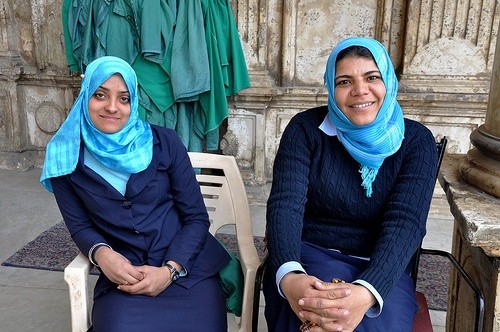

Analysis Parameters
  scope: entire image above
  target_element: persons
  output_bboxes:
[40,57,233,331]
[261,38,440,332]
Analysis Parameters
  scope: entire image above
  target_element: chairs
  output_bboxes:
[251,137,485,332]
[62,152,262,332]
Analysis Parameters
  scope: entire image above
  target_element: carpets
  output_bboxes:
[0,219,452,312]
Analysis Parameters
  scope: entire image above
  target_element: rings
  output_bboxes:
[123,281,128,285]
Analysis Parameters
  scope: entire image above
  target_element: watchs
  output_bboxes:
[164,263,180,281]
